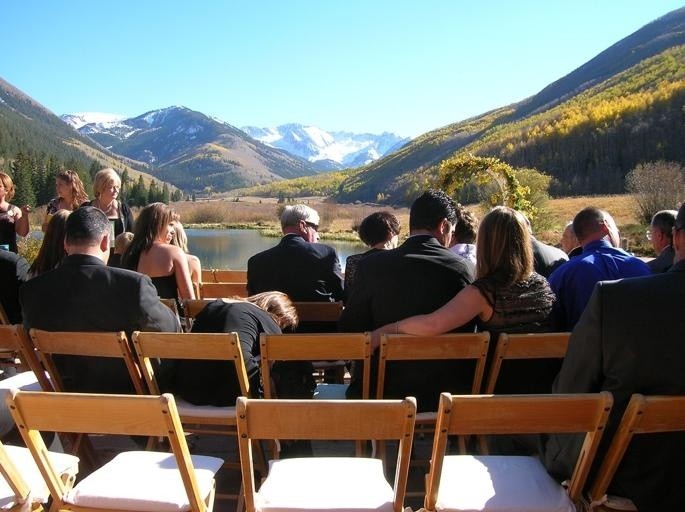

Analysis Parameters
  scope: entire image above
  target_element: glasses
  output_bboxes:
[645,230,656,240]
[305,222,318,232]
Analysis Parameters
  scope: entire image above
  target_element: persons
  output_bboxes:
[344,188,568,453]
[539,203,684,512]
[1,168,202,444]
[247,203,346,384]
[155,290,300,466]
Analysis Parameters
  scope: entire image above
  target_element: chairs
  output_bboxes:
[1,268,685,512]
